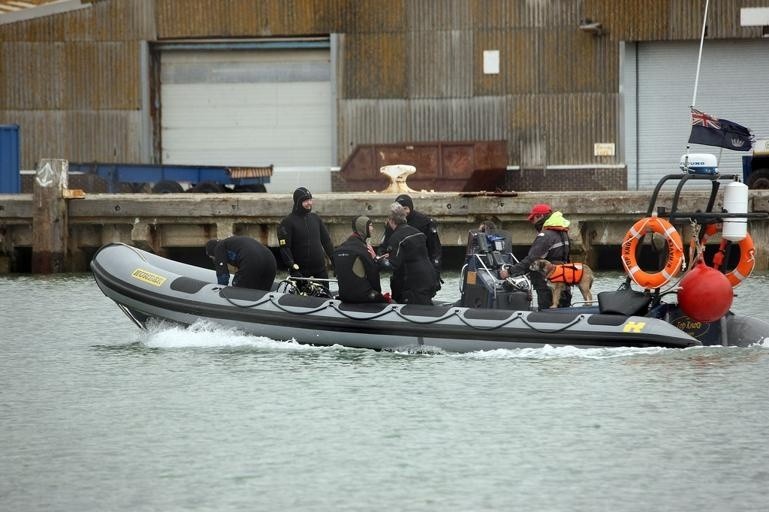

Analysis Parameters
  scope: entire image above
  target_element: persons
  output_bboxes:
[365,201,441,306]
[332,213,397,304]
[206,235,278,292]
[500,204,574,309]
[380,192,443,291]
[277,185,337,297]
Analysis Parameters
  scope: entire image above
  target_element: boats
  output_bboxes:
[89,240,768,358]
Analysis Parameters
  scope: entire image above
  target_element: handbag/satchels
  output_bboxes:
[598,290,651,316]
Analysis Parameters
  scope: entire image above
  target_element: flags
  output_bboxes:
[689,106,753,154]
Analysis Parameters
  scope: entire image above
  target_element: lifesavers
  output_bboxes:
[689,223,756,287]
[622,217,683,289]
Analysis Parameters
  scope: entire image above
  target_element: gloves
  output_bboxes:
[290,268,303,284]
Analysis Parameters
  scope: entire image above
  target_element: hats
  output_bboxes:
[293,188,312,213]
[352,215,370,239]
[395,195,413,213]
[205,241,217,256]
[528,205,552,219]
